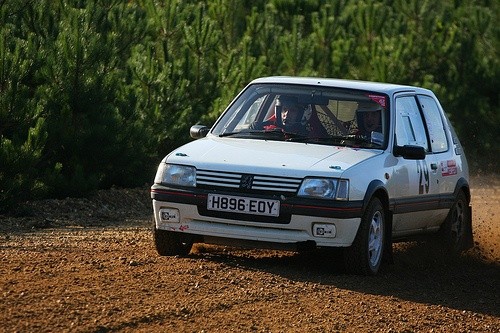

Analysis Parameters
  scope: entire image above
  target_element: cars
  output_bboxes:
[151,75,476,274]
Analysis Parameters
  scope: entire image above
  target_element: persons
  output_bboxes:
[249,94,305,140]
[347,101,381,142]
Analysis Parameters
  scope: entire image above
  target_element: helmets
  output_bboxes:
[356,101,382,112]
[274,95,312,127]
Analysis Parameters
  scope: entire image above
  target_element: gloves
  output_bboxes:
[248,121,264,135]
